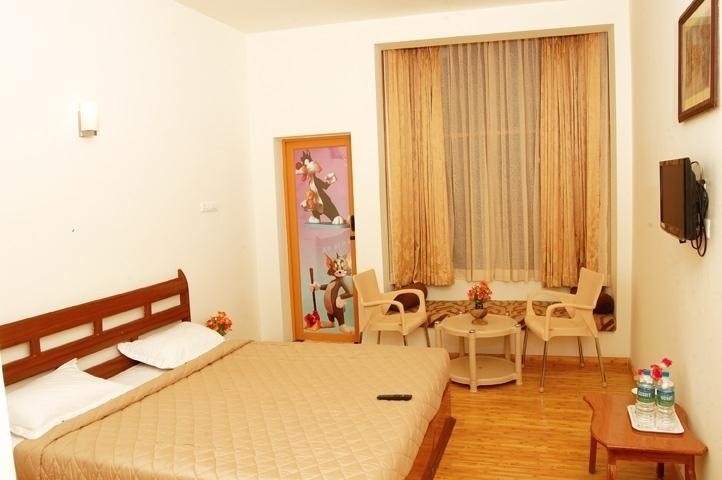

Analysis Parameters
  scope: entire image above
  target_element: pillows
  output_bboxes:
[6,358,129,441]
[117,321,226,369]
[390,281,428,312]
[571,285,614,315]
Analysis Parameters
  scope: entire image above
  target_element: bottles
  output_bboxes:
[635,368,654,427]
[656,371,675,432]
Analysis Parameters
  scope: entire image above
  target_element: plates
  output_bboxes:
[630,387,659,396]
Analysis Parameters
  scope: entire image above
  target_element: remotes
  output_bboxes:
[376,394,413,401]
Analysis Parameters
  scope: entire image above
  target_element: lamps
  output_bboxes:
[76,98,100,140]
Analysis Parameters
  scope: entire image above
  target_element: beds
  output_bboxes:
[1,268,456,480]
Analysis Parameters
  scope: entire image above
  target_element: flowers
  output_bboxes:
[464,278,493,304]
[636,356,672,381]
[205,310,233,337]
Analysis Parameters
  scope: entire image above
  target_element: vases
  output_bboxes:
[470,304,488,325]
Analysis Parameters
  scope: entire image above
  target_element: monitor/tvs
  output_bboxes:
[659,157,701,242]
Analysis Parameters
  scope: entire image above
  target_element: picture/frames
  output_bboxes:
[677,0,718,123]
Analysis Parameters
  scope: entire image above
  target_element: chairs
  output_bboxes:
[352,268,431,349]
[521,267,607,393]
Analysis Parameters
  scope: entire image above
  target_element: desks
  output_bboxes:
[582,392,708,480]
[433,313,523,393]
